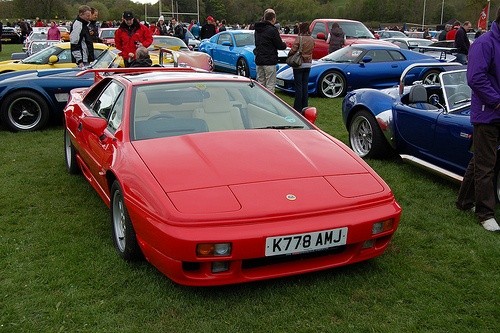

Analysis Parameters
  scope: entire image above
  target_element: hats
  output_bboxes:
[123,11,134,20]
[206,16,213,20]
[158,15,164,21]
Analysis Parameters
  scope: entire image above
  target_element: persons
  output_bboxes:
[367,18,472,65]
[454,7,500,232]
[135,14,254,45]
[326,23,347,54]
[130,46,152,67]
[61,20,73,26]
[252,8,287,94]
[0,16,44,52]
[97,19,122,28]
[114,11,154,68]
[287,22,314,114]
[46,21,61,41]
[69,5,95,67]
[86,7,101,42]
[275,22,300,33]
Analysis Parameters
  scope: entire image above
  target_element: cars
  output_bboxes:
[197,30,289,80]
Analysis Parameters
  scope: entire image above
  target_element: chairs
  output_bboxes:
[409,84,439,111]
[198,87,244,132]
[113,88,154,130]
[453,82,472,101]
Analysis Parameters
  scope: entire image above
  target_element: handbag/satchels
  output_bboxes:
[286,52,302,67]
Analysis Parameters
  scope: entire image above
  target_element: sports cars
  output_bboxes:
[0,22,214,133]
[341,64,475,183]
[275,31,476,100]
[62,70,401,288]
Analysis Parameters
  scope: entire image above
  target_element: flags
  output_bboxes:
[475,3,488,30]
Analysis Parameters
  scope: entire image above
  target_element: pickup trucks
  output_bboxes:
[279,19,400,62]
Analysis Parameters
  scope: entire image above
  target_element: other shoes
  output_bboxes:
[470,206,475,212]
[481,218,500,232]
